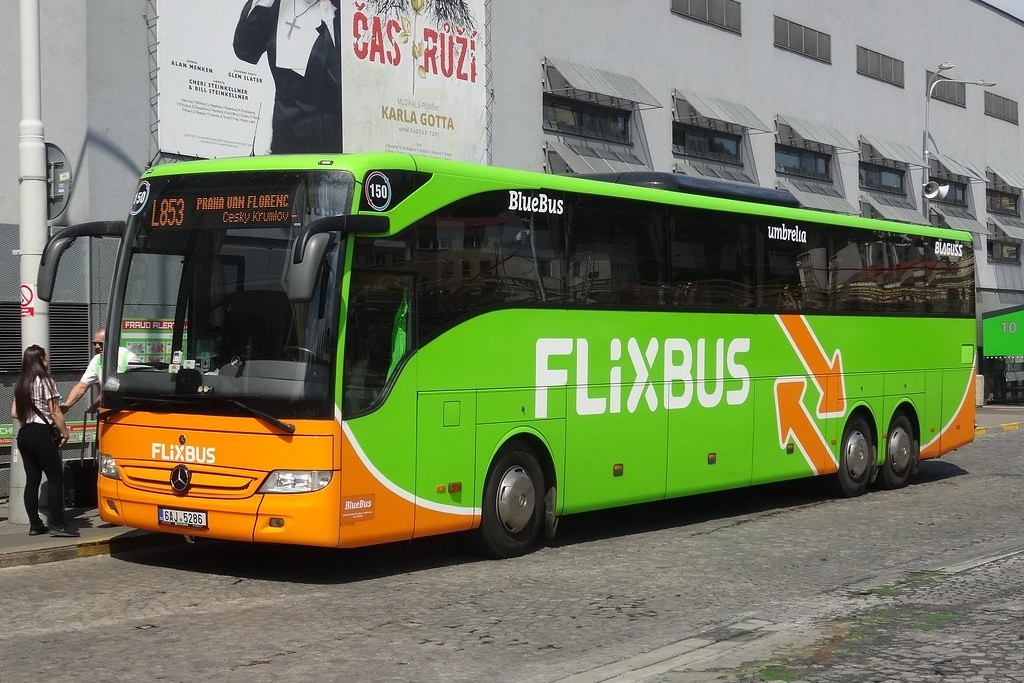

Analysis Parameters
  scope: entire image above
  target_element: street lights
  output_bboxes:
[924,61,997,227]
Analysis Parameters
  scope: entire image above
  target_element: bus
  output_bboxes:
[37,154,977,561]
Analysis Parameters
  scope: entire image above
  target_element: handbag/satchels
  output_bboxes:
[52,427,61,444]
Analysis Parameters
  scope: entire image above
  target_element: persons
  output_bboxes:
[10,344,81,537]
[58,325,144,414]
[233,0,343,155]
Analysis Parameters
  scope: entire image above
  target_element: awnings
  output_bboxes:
[778,177,861,214]
[986,165,1024,190]
[863,136,928,169]
[542,54,663,112]
[931,152,991,182]
[776,113,860,154]
[674,160,759,187]
[672,89,772,137]
[862,193,933,226]
[987,212,1024,239]
[935,206,993,234]
[544,139,652,174]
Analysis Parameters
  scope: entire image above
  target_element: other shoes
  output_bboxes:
[28,521,48,535]
[49,524,80,537]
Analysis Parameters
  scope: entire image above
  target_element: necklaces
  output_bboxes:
[285,0,320,39]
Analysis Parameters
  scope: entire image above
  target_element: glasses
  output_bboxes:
[92,340,103,347]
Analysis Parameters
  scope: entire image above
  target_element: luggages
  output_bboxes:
[64,410,99,508]
